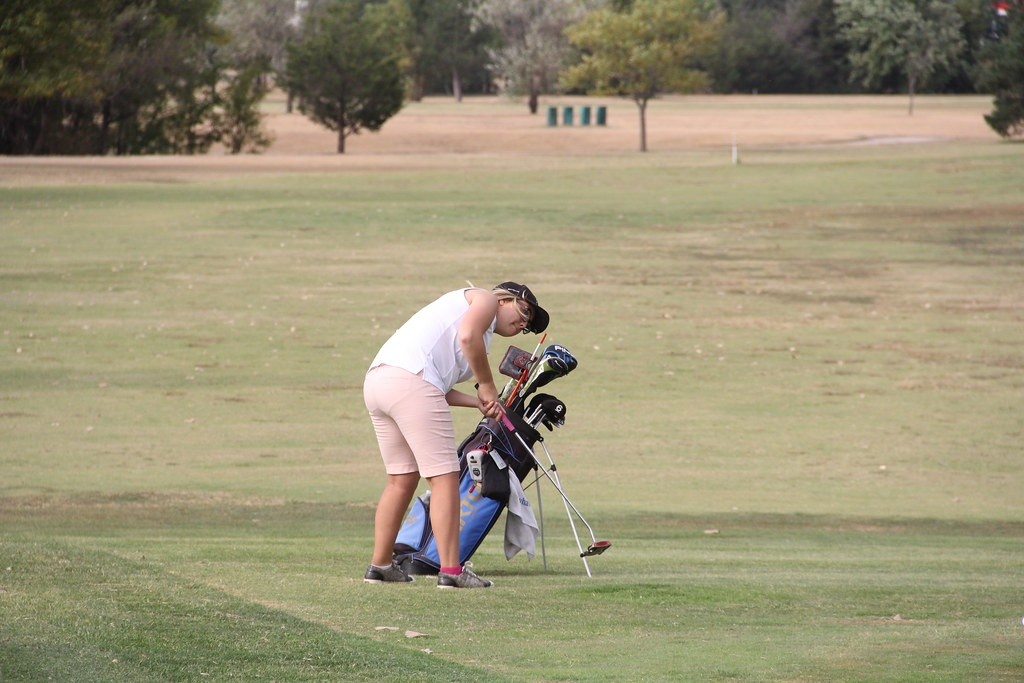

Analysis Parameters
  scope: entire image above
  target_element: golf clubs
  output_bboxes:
[474,381,612,558]
[517,344,580,432]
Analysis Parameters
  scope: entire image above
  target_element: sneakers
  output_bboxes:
[437,561,493,589]
[365,560,413,583]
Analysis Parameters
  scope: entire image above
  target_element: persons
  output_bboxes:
[364,281,549,589]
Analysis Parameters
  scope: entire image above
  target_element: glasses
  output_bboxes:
[516,300,532,334]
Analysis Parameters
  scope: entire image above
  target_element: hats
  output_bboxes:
[494,282,550,333]
